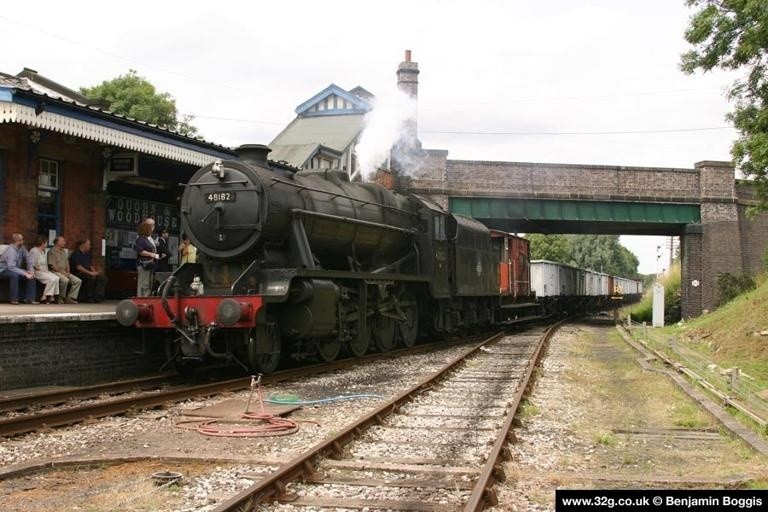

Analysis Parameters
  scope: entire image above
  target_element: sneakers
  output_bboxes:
[10,300,19,305]
[39,296,78,304]
[24,297,41,305]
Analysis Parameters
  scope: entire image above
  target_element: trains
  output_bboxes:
[117,144,645,375]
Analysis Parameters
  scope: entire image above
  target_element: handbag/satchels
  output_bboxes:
[142,259,156,270]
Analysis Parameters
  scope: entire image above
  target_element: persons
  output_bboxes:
[178,233,197,266]
[48,237,82,303]
[70,238,109,303]
[133,217,173,297]
[0,233,40,305]
[29,234,60,304]
